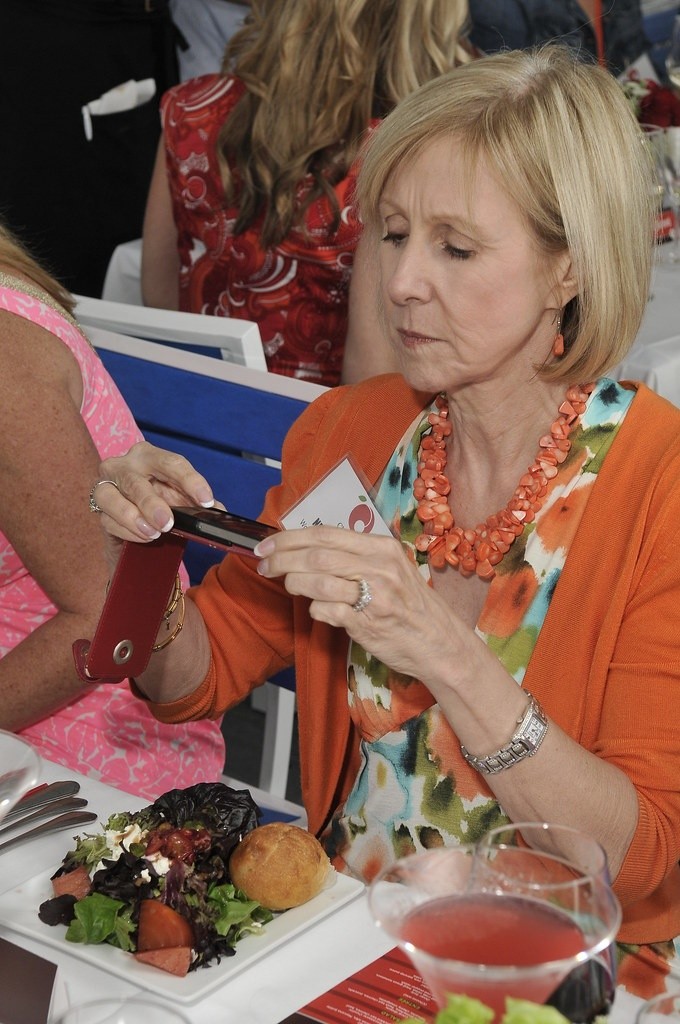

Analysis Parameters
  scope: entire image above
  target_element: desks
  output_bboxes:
[0,750,679,1024]
[98,204,677,406]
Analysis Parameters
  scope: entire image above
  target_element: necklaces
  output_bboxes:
[413,381,593,578]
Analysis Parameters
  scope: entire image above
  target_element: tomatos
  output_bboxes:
[135,947,191,978]
[52,866,92,900]
[137,898,194,952]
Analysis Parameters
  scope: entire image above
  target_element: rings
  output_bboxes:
[89,480,117,513]
[352,580,372,612]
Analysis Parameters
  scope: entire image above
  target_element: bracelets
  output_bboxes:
[104,574,185,652]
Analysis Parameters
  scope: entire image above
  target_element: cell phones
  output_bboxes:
[167,504,280,554]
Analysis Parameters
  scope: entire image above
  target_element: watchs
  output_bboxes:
[459,689,549,774]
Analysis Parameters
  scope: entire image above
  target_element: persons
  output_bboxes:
[90,47,680,958]
[143,0,476,387]
[473,0,680,92]
[0,229,226,1024]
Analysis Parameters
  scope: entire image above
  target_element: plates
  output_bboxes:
[1,852,366,1004]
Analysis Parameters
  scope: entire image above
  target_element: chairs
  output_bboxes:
[84,328,347,824]
[62,290,269,371]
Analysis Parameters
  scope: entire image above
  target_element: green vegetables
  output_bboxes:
[38,782,274,971]
[389,991,576,1024]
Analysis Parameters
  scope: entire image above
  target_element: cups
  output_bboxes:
[369,844,621,1014]
[468,820,618,1022]
[640,125,667,209]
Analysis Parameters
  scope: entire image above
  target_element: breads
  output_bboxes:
[229,822,332,913]
